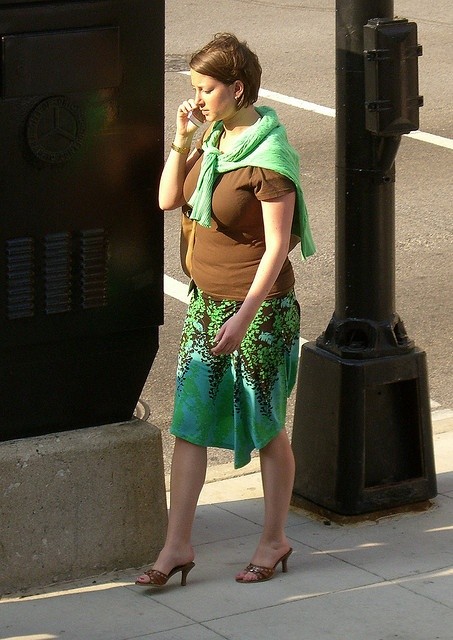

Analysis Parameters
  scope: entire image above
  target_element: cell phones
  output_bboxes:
[188,108,206,128]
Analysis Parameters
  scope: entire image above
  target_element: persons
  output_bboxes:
[135,32,317,587]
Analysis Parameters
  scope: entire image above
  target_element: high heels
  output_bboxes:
[135,562,195,587]
[235,547,293,583]
